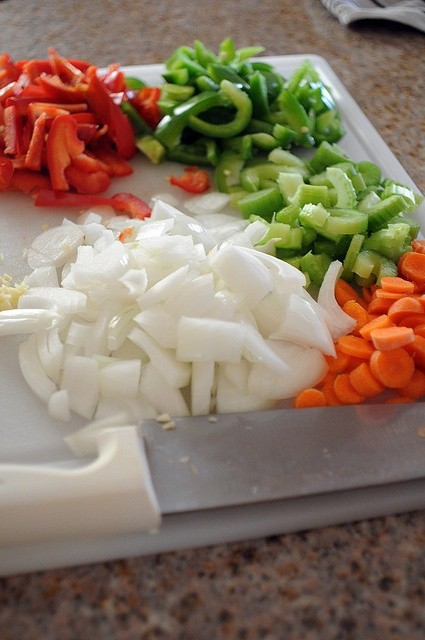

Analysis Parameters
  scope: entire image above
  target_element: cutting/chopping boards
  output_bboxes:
[0,53,423,582]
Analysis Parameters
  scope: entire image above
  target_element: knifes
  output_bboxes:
[1,403,424,525]
